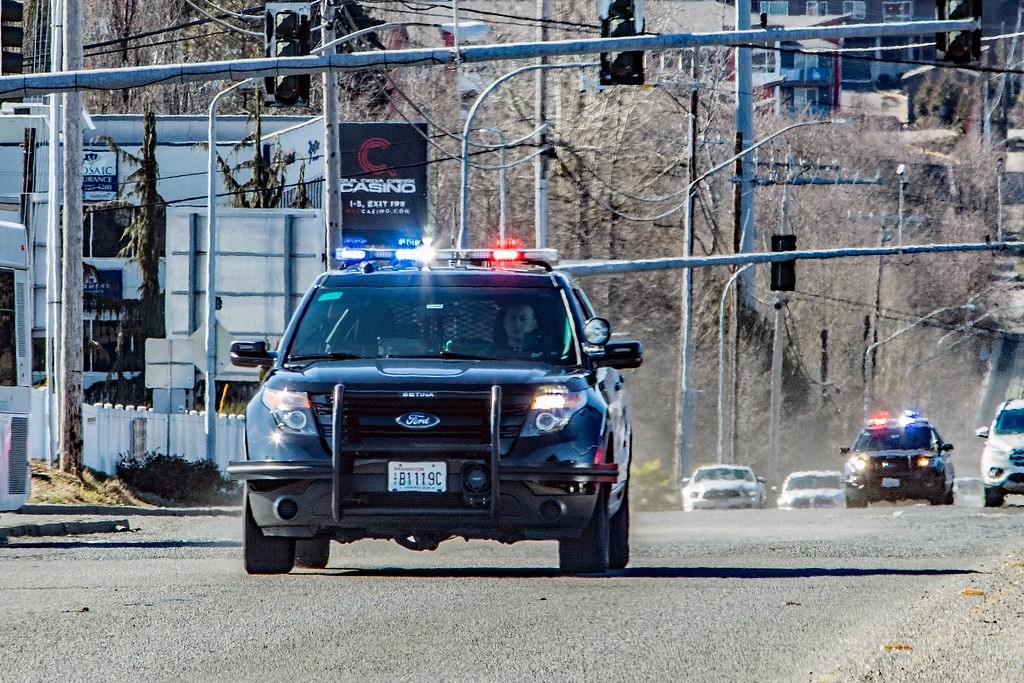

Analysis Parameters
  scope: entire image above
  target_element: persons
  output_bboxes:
[490,302,555,356]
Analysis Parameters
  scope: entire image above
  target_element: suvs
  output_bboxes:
[838,410,957,508]
[975,397,1024,508]
[225,245,643,578]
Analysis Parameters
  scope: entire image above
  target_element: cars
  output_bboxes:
[771,470,848,509]
[680,464,767,511]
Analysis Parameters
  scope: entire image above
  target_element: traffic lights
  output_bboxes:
[1,0,25,75]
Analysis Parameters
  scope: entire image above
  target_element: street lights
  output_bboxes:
[455,62,697,252]
[669,116,850,493]
[197,20,492,461]
